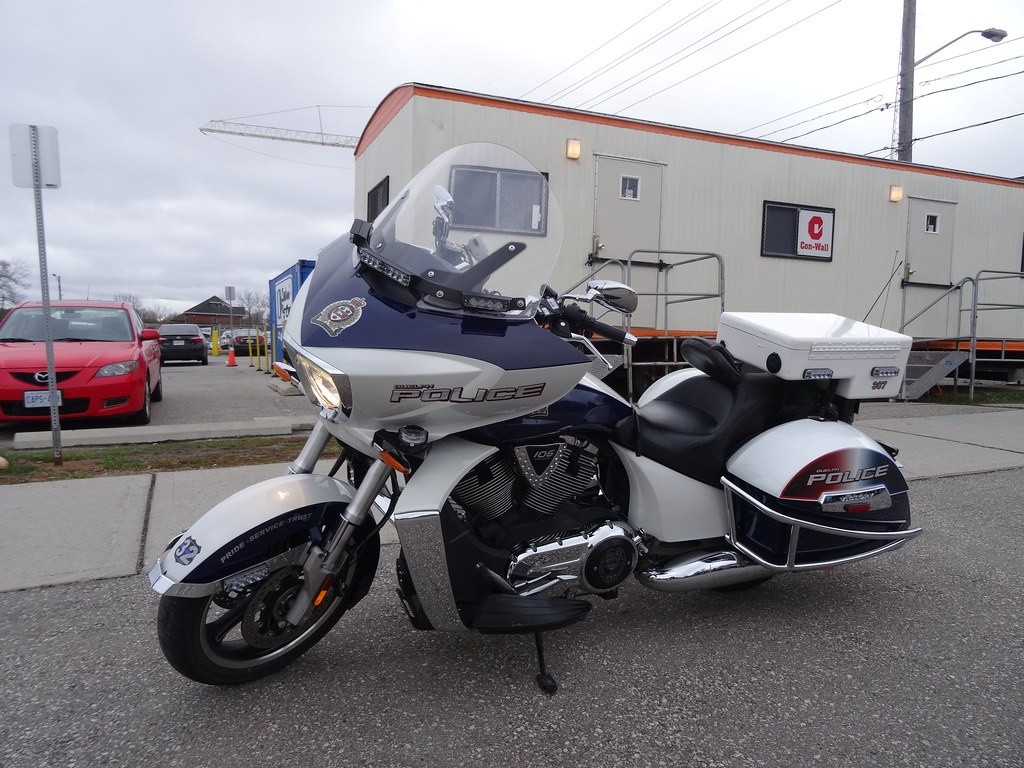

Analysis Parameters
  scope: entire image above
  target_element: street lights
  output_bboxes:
[896,0,1006,164]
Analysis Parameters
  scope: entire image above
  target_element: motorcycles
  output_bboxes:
[147,142,923,685]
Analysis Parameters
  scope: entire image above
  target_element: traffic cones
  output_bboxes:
[226,346,237,366]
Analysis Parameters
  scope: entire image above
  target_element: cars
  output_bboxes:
[219,329,272,357]
[0,300,164,424]
[157,323,212,366]
